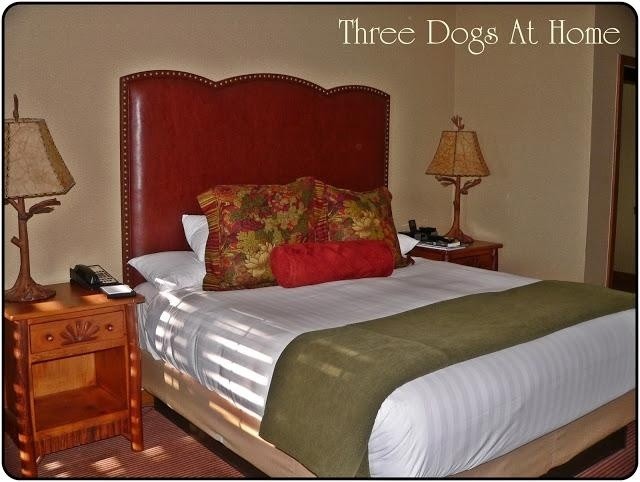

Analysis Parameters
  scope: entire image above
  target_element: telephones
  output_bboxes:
[70,264,122,290]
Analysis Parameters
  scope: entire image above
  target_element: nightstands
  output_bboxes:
[405,240,503,271]
[4,281,145,478]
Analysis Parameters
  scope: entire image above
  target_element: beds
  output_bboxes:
[134,252,635,478]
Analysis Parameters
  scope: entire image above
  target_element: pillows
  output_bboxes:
[127,178,421,293]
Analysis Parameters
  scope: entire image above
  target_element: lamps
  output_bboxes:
[4,93,76,303]
[425,115,490,244]
